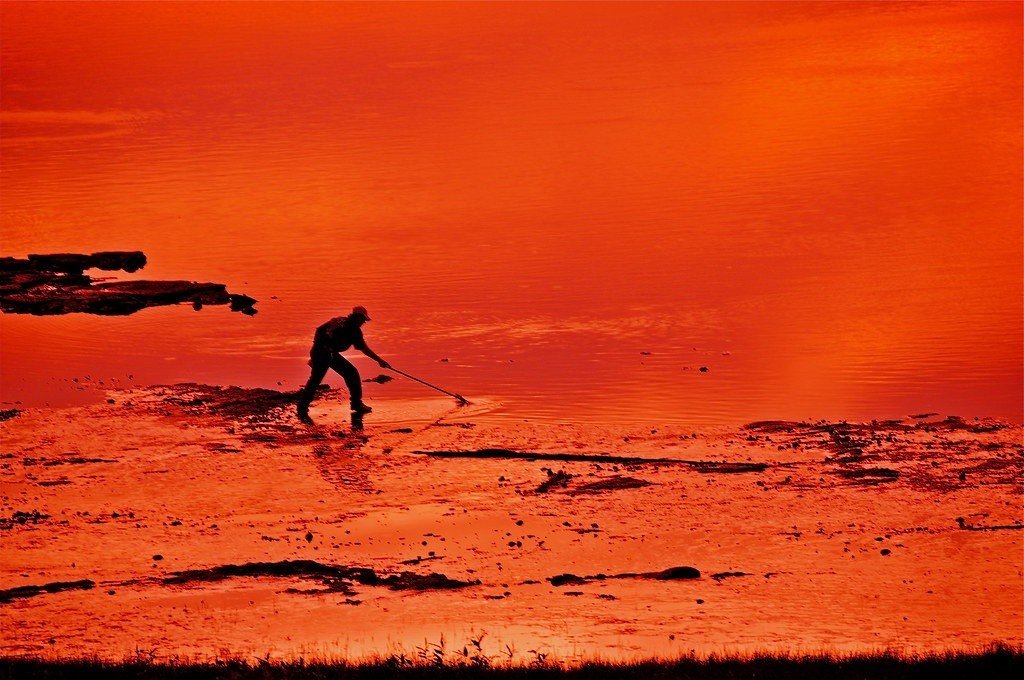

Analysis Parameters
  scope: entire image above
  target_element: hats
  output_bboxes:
[353,305,372,321]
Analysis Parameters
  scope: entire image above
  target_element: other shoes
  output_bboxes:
[298,410,310,420]
[351,402,372,411]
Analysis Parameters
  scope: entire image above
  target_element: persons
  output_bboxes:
[296,305,390,426]
